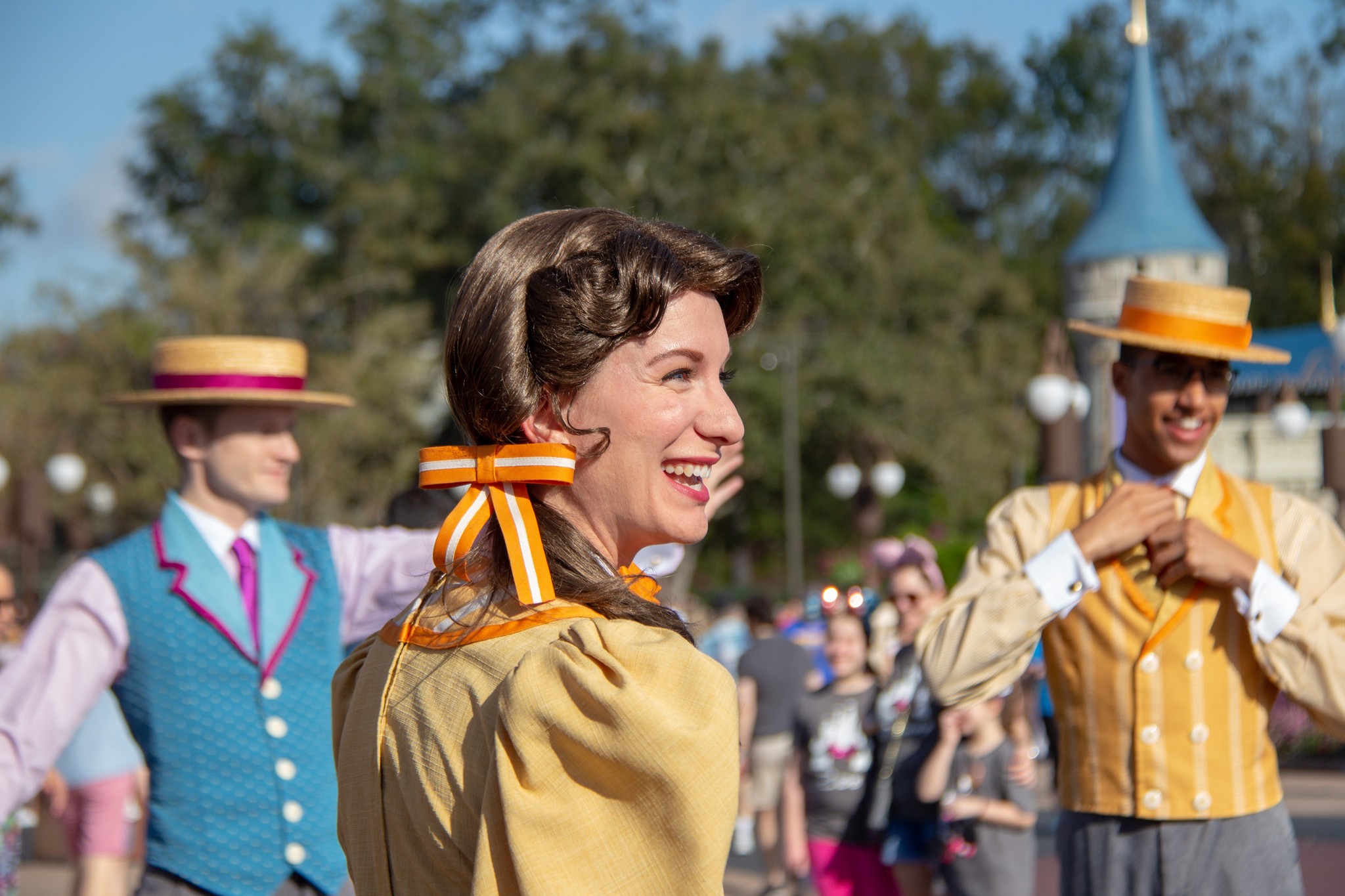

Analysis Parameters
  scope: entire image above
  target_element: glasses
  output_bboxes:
[1124,354,1239,395]
[0,597,17,608]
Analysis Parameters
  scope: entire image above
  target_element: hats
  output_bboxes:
[103,337,356,409]
[1067,275,1291,364]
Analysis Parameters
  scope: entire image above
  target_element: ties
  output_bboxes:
[234,538,260,650]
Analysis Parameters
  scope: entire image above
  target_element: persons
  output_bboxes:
[332,207,762,894]
[693,531,1059,896]
[0,565,140,896]
[913,278,1345,896]
[0,335,744,896]
